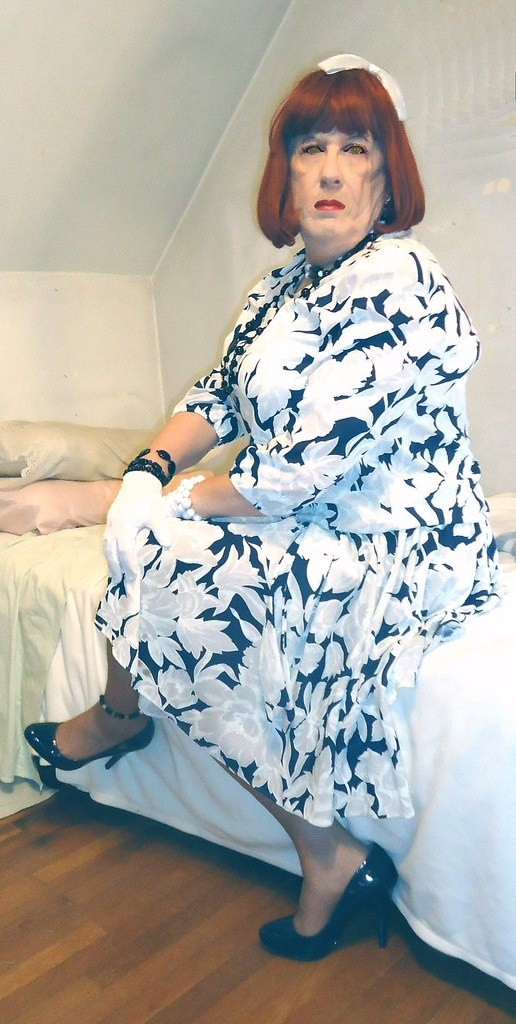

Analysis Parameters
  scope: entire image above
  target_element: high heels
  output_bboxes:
[258,842,398,960]
[25,715,154,770]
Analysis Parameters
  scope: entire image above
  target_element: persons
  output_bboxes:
[25,53,498,959]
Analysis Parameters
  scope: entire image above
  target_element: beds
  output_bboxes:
[0,491,515,994]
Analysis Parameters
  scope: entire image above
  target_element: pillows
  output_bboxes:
[0,480,124,538]
[0,420,154,481]
